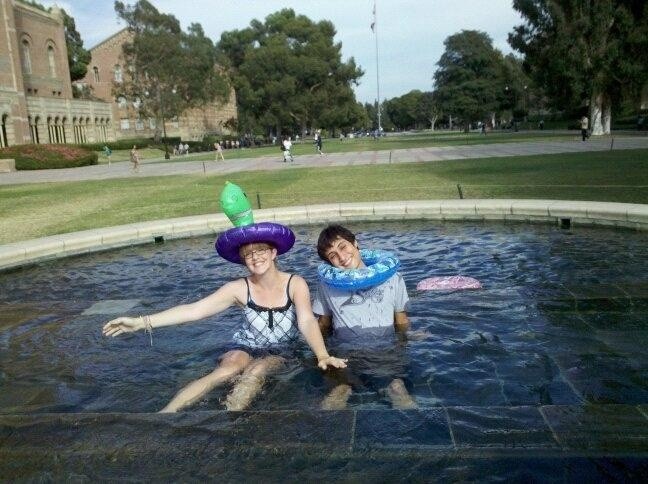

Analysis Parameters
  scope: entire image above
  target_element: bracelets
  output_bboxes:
[138,312,155,348]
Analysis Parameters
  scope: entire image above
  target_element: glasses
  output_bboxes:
[240,246,272,259]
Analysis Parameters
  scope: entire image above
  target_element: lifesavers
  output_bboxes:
[316,249,401,291]
[417,275,482,292]
[215,181,296,265]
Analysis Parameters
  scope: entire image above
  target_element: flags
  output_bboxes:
[371,7,375,33]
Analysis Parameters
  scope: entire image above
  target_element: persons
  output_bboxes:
[212,142,224,162]
[312,132,325,156]
[127,143,143,173]
[220,137,245,152]
[280,136,295,162]
[101,145,113,167]
[310,222,431,413]
[99,236,350,415]
[171,141,191,157]
[580,113,592,141]
